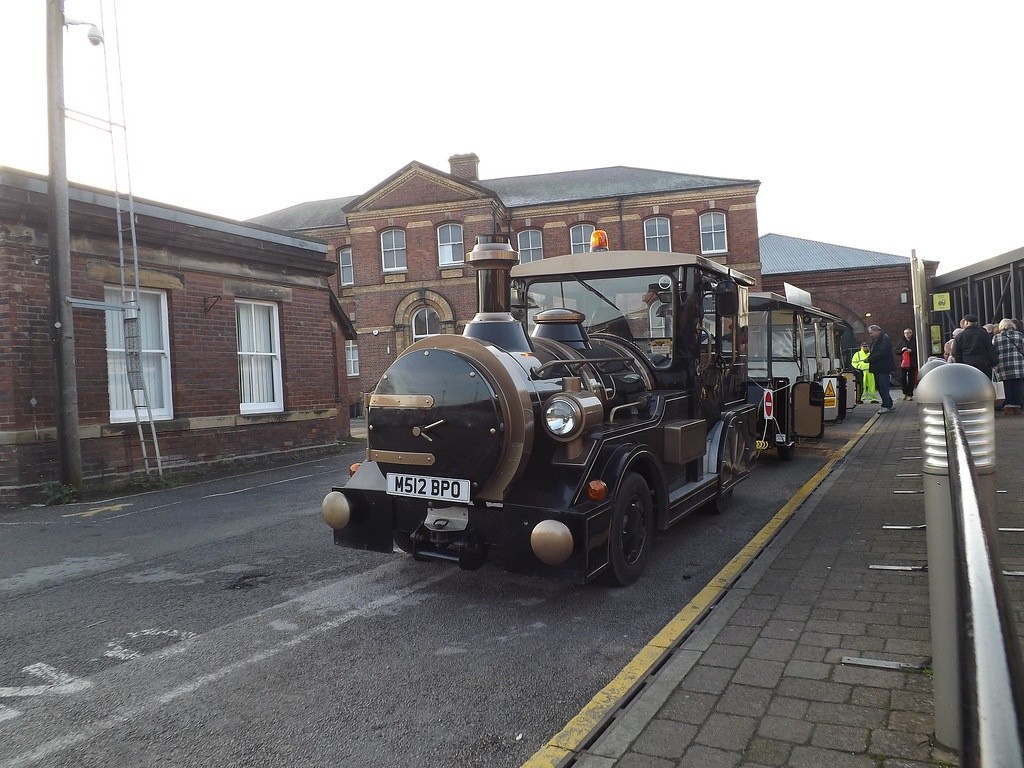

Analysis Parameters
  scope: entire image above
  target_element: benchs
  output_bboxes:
[747,354,840,403]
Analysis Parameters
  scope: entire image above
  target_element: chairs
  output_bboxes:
[583,306,634,343]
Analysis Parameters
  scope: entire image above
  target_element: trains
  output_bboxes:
[322,229,865,590]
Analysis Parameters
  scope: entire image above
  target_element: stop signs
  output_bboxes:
[765,391,774,418]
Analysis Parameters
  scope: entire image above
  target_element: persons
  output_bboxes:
[863,325,895,414]
[851,341,879,402]
[894,327,917,401]
[942,315,1024,414]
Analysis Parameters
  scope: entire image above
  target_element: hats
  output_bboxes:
[964,314,978,322]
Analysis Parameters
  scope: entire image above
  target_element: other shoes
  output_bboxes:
[902,394,906,400]
[1002,404,1013,415]
[858,401,863,404]
[906,396,913,401]
[877,407,889,414]
[871,400,879,403]
[1013,405,1021,414]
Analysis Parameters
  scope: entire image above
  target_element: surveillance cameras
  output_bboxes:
[88,27,103,46]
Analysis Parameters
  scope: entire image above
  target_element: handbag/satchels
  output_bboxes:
[991,369,1006,400]
[901,352,910,368]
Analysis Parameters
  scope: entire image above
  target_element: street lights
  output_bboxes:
[45,0,107,488]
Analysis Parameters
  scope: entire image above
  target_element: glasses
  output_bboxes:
[864,346,867,347]
